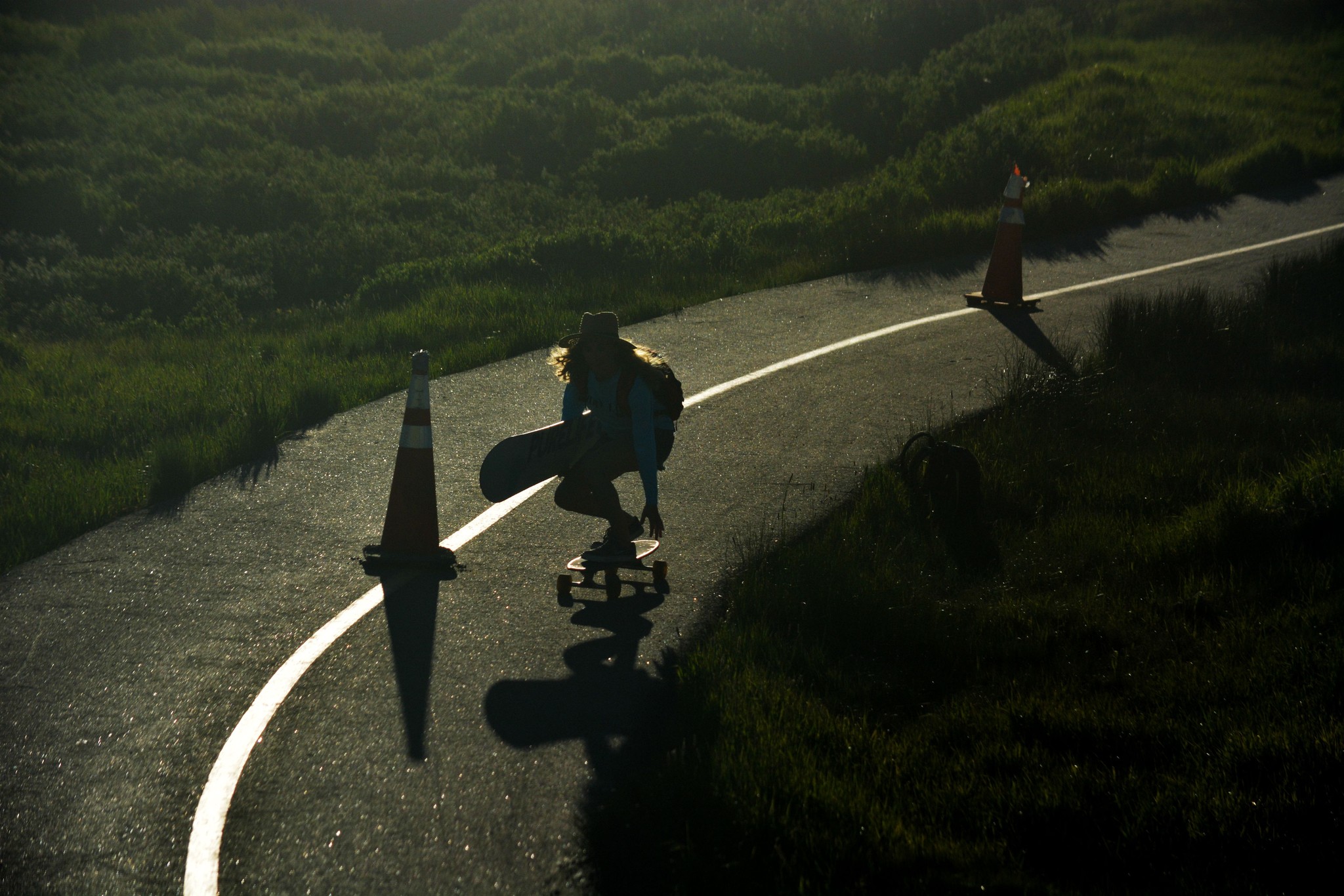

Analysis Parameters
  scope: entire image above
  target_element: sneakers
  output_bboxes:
[582,539,636,562]
[590,516,644,549]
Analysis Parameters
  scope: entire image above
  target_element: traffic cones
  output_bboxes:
[964,161,1043,316]
[349,349,462,576]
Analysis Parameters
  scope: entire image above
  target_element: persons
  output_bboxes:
[553,312,684,555]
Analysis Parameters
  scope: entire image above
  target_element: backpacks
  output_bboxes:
[576,352,684,422]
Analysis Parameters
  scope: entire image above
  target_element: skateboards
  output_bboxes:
[555,538,668,597]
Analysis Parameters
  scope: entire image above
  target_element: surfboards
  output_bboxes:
[479,408,599,504]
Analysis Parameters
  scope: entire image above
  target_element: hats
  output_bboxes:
[558,312,637,352]
